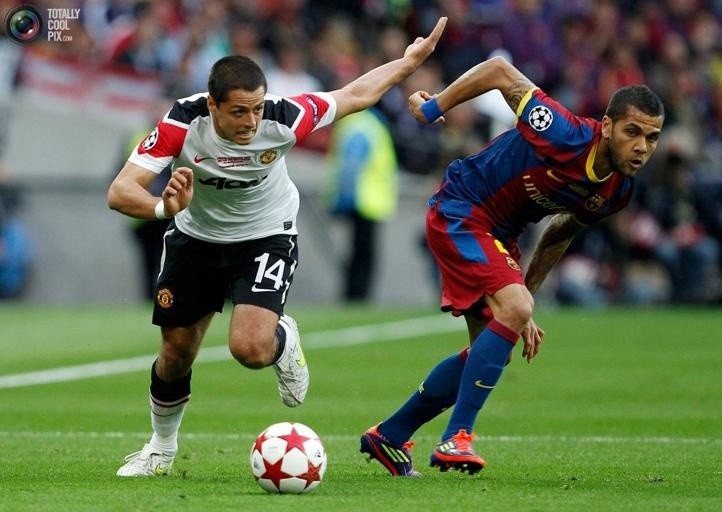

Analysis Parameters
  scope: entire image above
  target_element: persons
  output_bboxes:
[65,16,452,490]
[316,102,400,304]
[0,0,720,312]
[359,52,669,479]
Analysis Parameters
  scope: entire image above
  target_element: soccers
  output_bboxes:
[250,421,327,494]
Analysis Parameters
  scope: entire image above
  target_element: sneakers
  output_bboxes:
[359,422,423,479]
[429,428,488,475]
[272,314,310,408]
[115,443,174,477]
